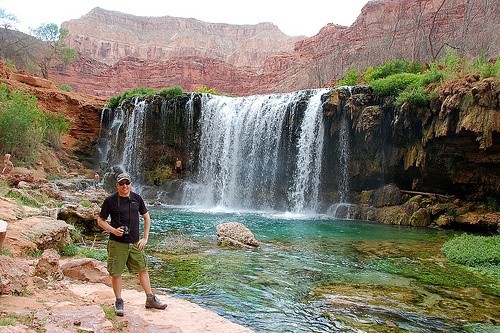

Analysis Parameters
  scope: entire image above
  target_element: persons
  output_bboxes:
[93,172,100,189]
[97,172,167,316]
[175,157,181,179]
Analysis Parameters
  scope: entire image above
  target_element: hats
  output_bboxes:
[117,173,130,182]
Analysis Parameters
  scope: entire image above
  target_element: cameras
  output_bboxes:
[119,226,131,238]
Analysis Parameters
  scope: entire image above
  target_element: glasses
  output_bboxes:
[118,181,130,186]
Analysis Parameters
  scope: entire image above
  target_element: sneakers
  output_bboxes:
[115,298,124,315]
[145,294,168,309]
